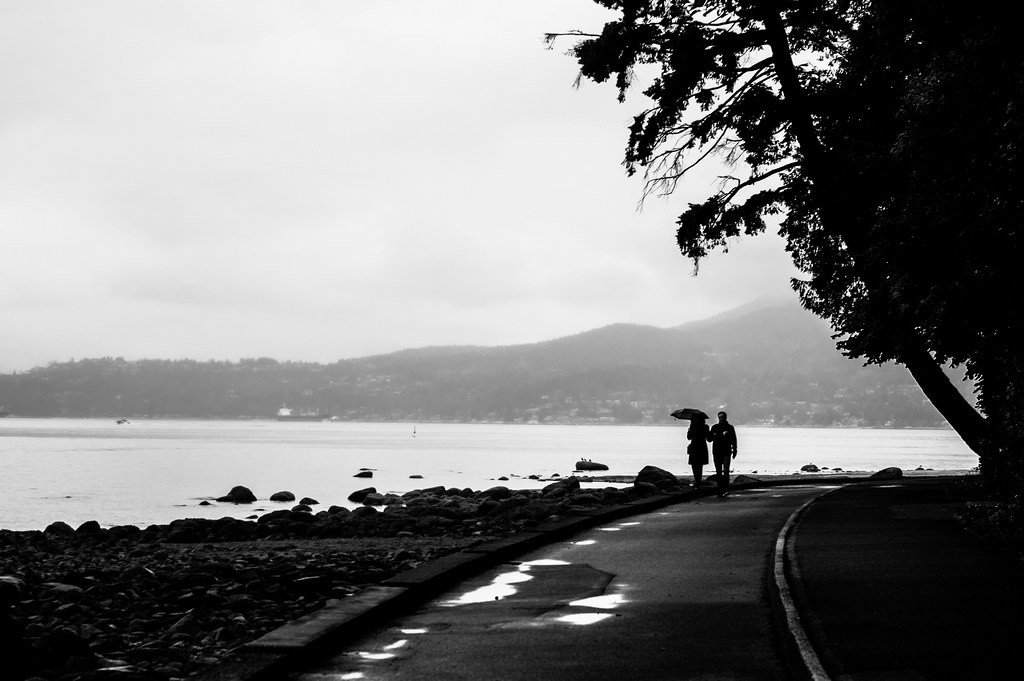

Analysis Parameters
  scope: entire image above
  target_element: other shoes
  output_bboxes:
[717,490,730,498]
[694,482,700,491]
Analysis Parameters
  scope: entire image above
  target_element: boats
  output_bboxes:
[277,408,329,422]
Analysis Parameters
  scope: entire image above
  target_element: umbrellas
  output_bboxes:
[672,408,709,419]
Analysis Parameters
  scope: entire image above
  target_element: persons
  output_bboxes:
[710,411,737,496]
[687,414,716,487]
